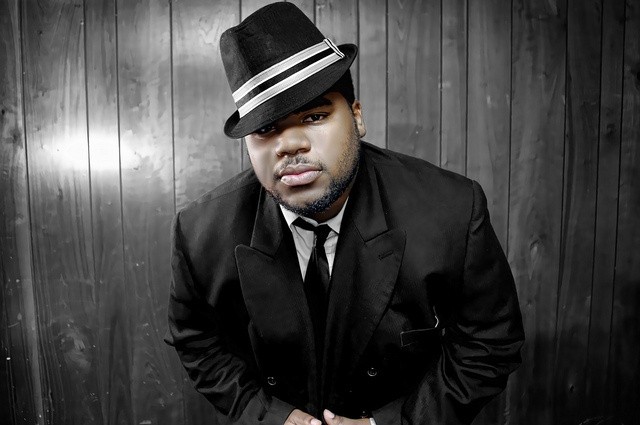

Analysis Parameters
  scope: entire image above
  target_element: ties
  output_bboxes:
[292,217,331,383]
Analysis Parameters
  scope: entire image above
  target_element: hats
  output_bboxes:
[219,1,357,139]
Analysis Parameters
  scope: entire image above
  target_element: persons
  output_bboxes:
[164,2,525,424]
